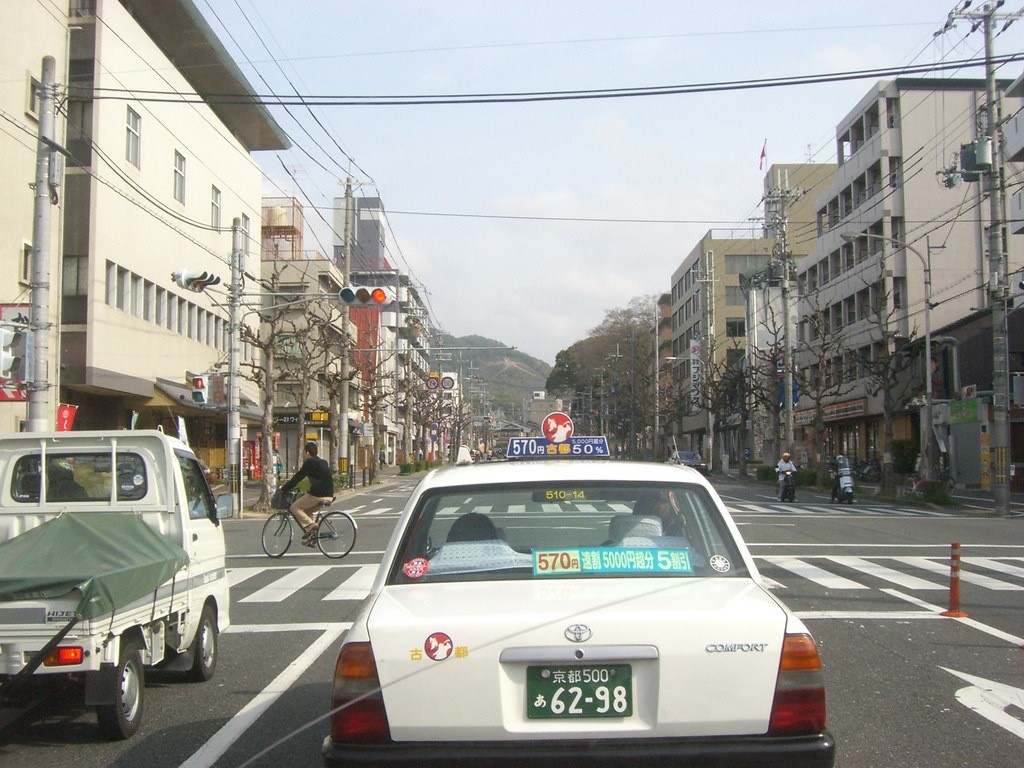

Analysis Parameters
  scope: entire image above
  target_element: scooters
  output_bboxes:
[828,457,855,505]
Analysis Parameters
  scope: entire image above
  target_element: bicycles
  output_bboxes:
[902,467,957,498]
[262,487,358,559]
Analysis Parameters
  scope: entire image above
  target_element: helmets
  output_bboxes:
[836,454,844,464]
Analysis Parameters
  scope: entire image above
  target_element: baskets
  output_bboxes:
[270,490,293,509]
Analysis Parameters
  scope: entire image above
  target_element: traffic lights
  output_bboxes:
[191,374,209,405]
[338,286,393,306]
[171,269,221,294]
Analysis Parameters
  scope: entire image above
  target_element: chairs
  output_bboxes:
[611,536,705,566]
[608,512,667,542]
[116,471,146,499]
[427,537,529,573]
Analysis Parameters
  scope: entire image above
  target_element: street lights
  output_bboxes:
[665,356,713,473]
[841,231,934,478]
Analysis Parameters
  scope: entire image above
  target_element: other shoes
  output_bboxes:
[301,538,317,548]
[302,522,318,539]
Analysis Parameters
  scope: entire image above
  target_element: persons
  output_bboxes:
[448,513,498,541]
[632,490,674,534]
[774,452,798,502]
[278,442,334,547]
[829,452,857,504]
[379,448,386,469]
[275,451,282,479]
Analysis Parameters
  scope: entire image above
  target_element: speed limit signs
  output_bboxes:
[441,376,454,390]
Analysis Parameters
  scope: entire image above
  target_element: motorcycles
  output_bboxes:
[775,463,801,503]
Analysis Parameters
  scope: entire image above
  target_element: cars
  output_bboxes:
[664,451,708,477]
[320,411,837,768]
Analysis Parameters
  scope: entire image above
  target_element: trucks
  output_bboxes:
[1,429,234,741]
